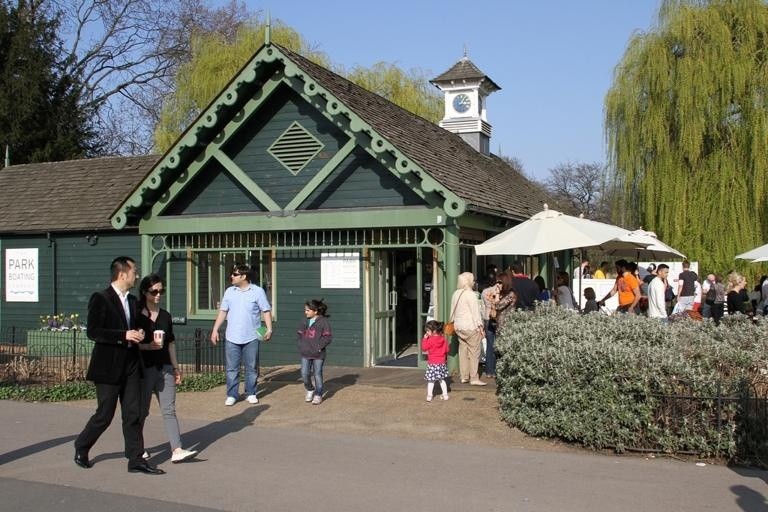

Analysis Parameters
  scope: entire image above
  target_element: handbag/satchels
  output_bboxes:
[705,288,716,305]
[665,284,674,302]
[445,321,455,336]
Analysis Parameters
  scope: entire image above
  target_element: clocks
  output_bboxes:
[453,94,471,113]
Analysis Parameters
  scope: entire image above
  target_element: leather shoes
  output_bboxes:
[75,448,89,468]
[128,459,163,475]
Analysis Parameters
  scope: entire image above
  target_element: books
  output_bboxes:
[255,326,267,338]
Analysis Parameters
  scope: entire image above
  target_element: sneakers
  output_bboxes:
[171,449,198,463]
[312,395,322,404]
[471,380,486,386]
[247,394,258,403]
[305,390,314,401]
[226,397,236,406]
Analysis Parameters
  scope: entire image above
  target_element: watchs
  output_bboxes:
[267,329,272,333]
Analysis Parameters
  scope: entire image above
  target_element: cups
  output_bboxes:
[153,329,165,349]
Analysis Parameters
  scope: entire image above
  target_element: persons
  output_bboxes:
[573,257,640,315]
[481,261,548,378]
[210,263,274,407]
[401,261,433,336]
[450,272,487,386]
[635,263,675,315]
[73,257,165,476]
[134,273,199,464]
[295,298,333,406]
[694,273,726,315]
[553,271,575,309]
[672,259,699,314]
[421,320,449,402]
[727,270,767,315]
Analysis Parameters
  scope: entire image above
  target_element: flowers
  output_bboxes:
[39,314,87,332]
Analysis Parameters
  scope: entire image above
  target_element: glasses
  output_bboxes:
[233,272,241,277]
[148,289,166,296]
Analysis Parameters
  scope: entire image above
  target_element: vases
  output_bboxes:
[27,330,95,357]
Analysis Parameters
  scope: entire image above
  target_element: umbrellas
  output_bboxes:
[474,202,768,315]
